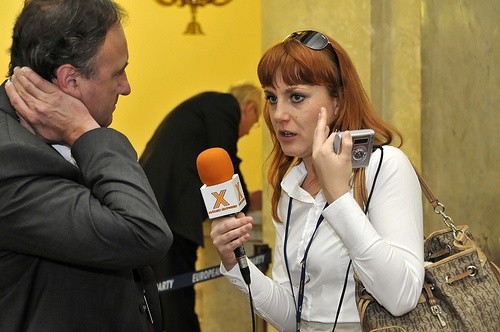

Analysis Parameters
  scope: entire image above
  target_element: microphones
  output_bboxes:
[197,148,252,285]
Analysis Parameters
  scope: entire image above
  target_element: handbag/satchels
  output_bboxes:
[352,144,499,332]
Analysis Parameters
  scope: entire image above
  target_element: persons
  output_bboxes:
[138,84,262,332]
[211,30,425,332]
[0,0,173,332]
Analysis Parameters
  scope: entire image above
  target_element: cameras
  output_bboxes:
[334,129,375,168]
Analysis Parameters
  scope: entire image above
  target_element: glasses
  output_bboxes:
[252,107,260,128]
[281,30,337,55]
